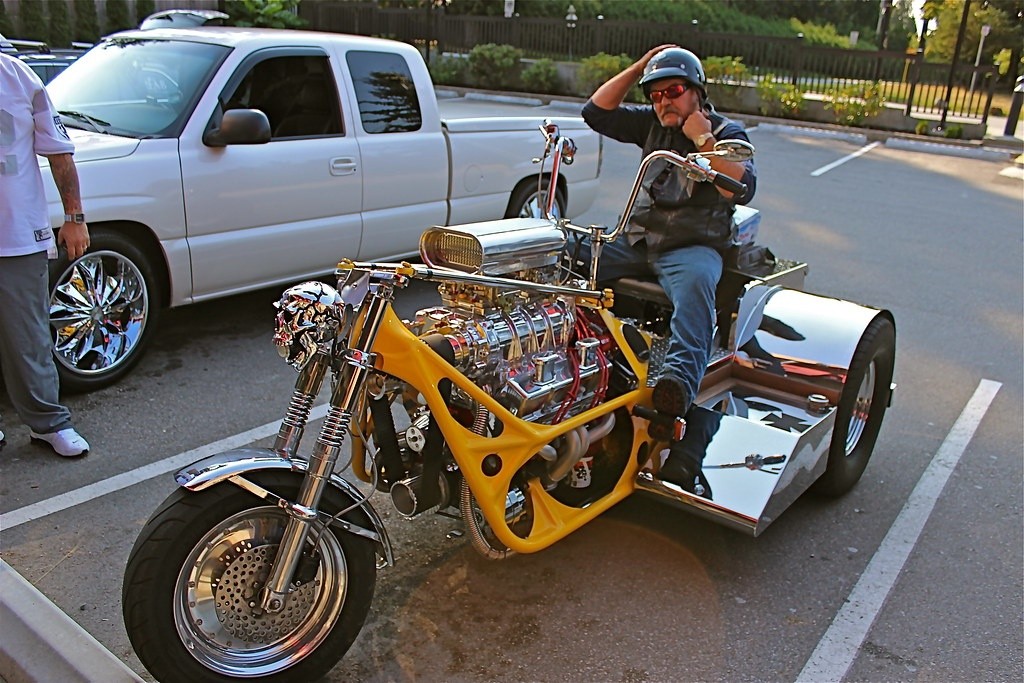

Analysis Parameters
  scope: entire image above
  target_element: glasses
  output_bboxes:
[650,81,692,102]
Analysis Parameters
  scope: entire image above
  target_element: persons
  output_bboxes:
[2,45,96,457]
[548,44,763,446]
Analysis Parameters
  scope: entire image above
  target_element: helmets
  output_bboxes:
[637,46,707,98]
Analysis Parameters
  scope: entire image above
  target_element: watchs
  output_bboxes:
[694,130,714,149]
[63,212,85,225]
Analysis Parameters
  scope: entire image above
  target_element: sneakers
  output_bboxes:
[30,428,90,458]
[647,377,692,442]
[0,428,6,452]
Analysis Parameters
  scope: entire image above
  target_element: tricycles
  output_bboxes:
[120,113,903,683]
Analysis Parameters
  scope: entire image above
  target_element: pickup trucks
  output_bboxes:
[27,25,604,394]
